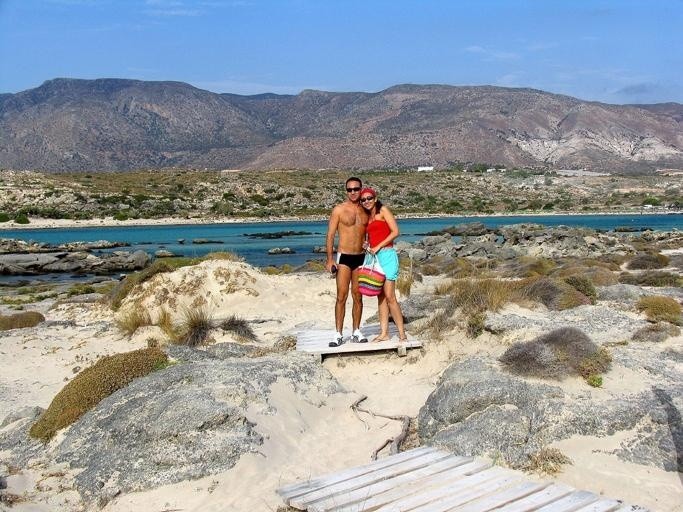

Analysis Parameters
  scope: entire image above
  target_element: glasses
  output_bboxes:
[359,196,373,202]
[346,187,360,192]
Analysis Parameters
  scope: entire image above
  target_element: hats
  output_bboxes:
[359,188,377,197]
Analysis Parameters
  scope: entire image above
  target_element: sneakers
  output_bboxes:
[327,332,343,347]
[349,329,367,343]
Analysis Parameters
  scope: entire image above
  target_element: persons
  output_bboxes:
[323,176,369,348]
[356,187,408,344]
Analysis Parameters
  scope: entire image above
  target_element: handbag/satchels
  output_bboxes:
[355,251,385,298]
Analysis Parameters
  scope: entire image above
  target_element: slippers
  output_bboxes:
[370,334,386,344]
[397,336,408,344]
